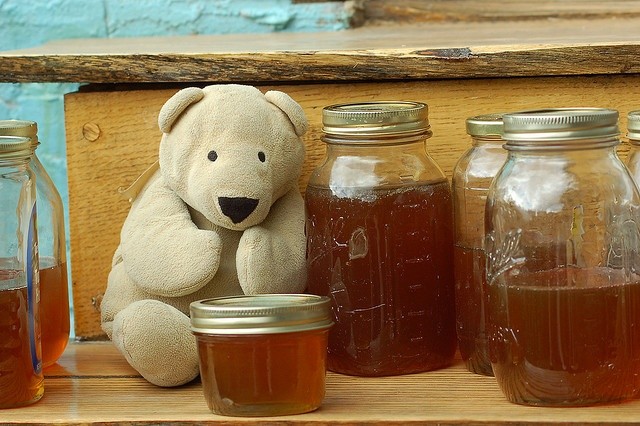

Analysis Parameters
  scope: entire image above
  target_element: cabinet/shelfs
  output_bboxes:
[0,19,640,426]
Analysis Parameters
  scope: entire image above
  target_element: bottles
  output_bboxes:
[481,106,639,406]
[0,136,46,410]
[0,117,70,366]
[299,98,462,375]
[450,106,572,378]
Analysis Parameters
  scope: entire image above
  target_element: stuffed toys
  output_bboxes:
[99,84,313,387]
